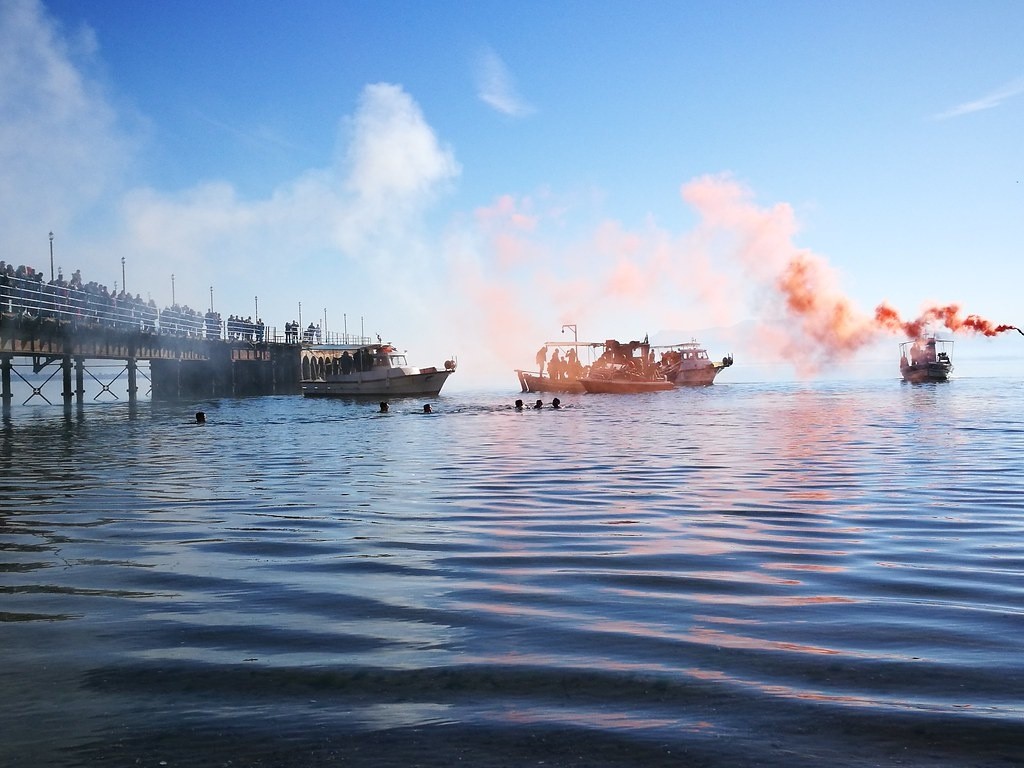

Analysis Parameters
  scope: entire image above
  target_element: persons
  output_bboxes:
[534,400,543,408]
[0,261,158,333]
[552,397,560,408]
[380,402,389,412]
[285,320,299,344]
[424,404,433,413]
[622,349,661,381]
[196,412,207,423]
[515,400,523,408]
[205,308,222,339]
[304,322,322,345]
[660,351,679,366]
[160,304,204,337]
[338,349,374,375]
[536,346,577,379]
[228,314,264,342]
[910,343,918,366]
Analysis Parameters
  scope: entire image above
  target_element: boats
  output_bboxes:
[899,328,952,383]
[578,334,677,392]
[652,338,733,386]
[300,335,456,399]
[514,324,684,394]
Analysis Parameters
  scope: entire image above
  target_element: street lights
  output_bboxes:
[344,313,347,344]
[121,256,127,293]
[210,286,214,313]
[298,302,302,339]
[171,273,175,306]
[361,316,364,345]
[49,230,55,281]
[324,308,327,343]
[255,296,258,324]
